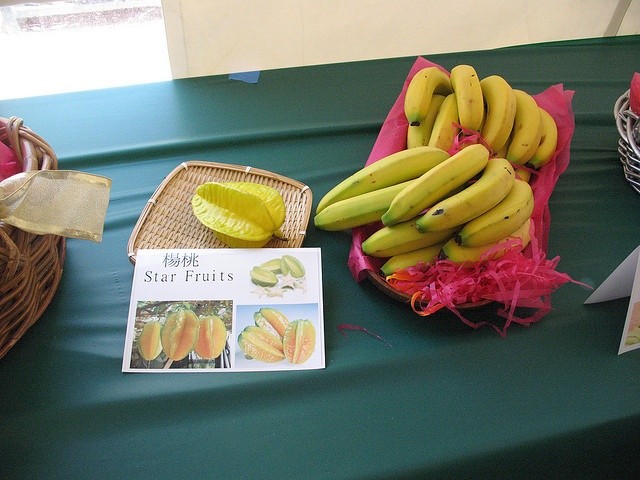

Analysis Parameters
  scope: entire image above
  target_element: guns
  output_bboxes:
[404,65,559,183]
[313,143,535,277]
